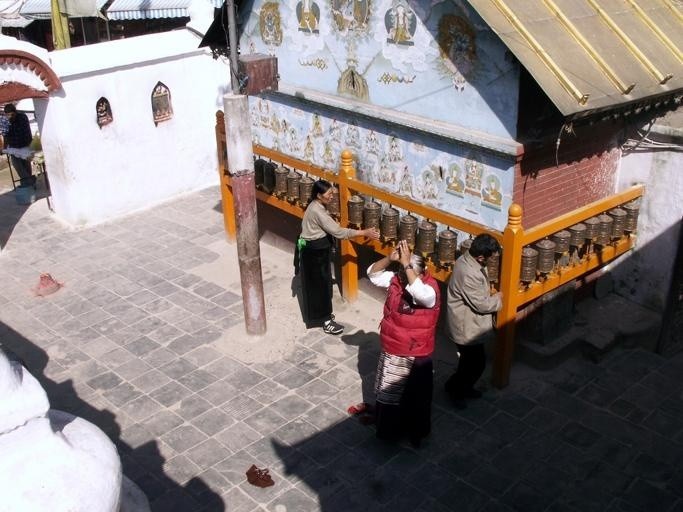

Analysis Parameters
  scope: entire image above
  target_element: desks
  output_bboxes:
[4,147,52,213]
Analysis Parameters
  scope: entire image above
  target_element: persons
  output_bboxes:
[3,103,39,187]
[293,178,379,334]
[367,238,438,449]
[445,233,504,410]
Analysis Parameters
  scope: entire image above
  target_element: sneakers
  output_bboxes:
[322,314,344,334]
